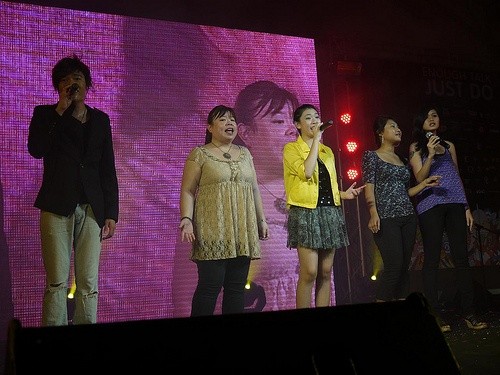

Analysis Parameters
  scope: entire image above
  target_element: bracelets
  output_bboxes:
[180,216,192,222]
[257,220,268,223]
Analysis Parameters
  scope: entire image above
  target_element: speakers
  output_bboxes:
[3,291,465,375]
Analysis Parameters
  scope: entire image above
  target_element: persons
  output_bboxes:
[407,105,488,332]
[27,55,121,326]
[226,80,338,312]
[176,106,269,317]
[284,103,366,309]
[362,116,444,303]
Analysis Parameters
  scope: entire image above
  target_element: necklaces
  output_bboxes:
[210,140,233,159]
[256,179,288,214]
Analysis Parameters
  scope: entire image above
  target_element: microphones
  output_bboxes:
[67,82,79,98]
[425,131,451,149]
[319,119,334,132]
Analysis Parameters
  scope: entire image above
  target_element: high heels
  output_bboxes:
[440,325,451,332]
[464,319,487,329]
